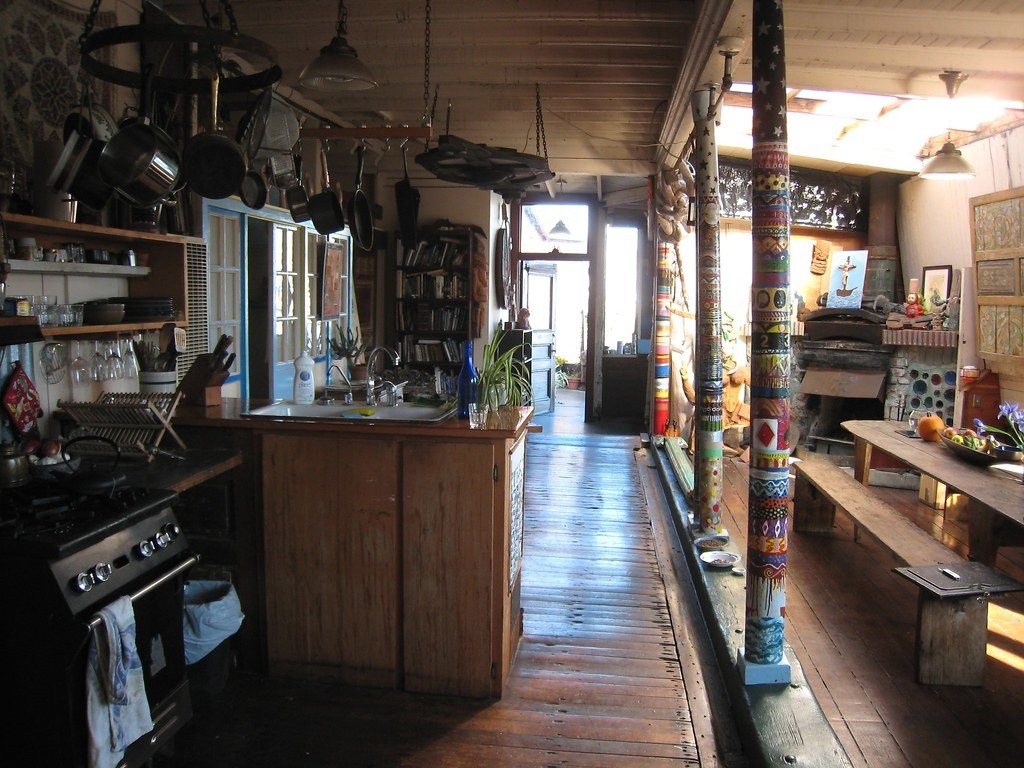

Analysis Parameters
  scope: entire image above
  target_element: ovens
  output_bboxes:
[0,547,195,767]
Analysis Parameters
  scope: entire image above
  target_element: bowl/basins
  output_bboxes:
[993,444,1023,462]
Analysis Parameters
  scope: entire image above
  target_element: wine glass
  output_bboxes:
[70,339,138,382]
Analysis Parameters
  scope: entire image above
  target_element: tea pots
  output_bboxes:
[0,439,31,487]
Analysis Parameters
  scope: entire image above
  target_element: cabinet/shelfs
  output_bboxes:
[0,211,189,344]
[392,224,488,382]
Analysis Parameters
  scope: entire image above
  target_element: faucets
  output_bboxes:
[366,345,400,405]
[327,365,353,404]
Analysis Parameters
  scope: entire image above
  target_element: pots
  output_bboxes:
[30,31,371,247]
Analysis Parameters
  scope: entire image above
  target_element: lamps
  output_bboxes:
[547,177,571,237]
[296,0,379,93]
[917,127,981,180]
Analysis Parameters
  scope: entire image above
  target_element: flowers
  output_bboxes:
[973,401,1024,455]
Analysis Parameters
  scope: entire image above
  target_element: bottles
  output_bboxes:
[293,350,315,405]
[909,409,929,434]
[456,341,479,419]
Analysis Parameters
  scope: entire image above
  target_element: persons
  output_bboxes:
[838,262,855,285]
[680,321,750,458]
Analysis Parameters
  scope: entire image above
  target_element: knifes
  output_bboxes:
[206,335,237,372]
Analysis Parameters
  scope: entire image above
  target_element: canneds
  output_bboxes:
[4,297,29,316]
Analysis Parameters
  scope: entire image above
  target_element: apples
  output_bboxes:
[944,426,989,452]
[22,439,58,456]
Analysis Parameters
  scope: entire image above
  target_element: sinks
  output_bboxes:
[373,406,440,420]
[263,405,348,418]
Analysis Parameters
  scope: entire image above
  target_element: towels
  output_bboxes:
[84,594,154,768]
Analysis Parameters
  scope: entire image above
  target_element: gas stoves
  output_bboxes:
[0,435,189,619]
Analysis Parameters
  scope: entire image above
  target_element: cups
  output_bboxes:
[467,403,489,430]
[10,294,85,329]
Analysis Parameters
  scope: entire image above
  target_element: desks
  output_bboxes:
[841,419,1024,574]
[240,405,536,700]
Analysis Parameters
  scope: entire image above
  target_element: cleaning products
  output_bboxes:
[293,351,315,404]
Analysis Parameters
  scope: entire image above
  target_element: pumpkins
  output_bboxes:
[918,411,945,442]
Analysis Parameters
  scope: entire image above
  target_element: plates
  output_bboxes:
[936,428,1006,465]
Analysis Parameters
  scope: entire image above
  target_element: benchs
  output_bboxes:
[793,460,988,687]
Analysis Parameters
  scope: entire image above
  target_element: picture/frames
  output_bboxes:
[921,265,954,312]
[319,241,343,318]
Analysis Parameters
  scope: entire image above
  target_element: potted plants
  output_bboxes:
[325,324,368,380]
[468,327,536,428]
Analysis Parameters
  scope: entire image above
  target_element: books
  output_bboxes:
[396,235,467,401]
